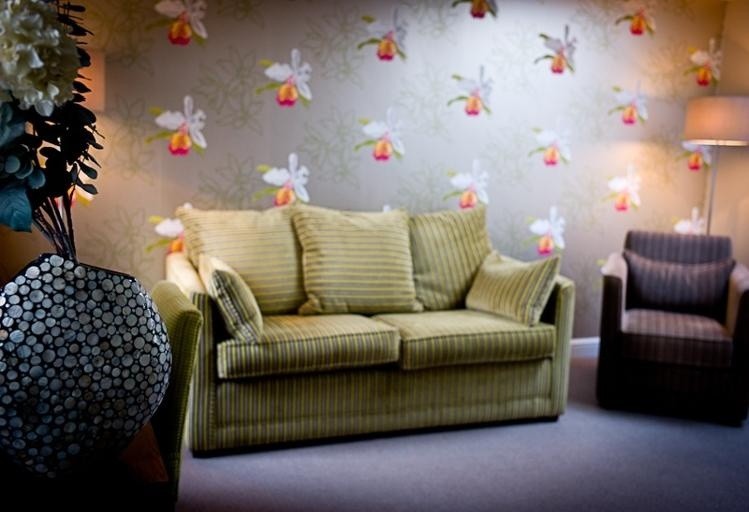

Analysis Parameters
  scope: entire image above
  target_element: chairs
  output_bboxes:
[596,229,749,428]
[150,278,204,504]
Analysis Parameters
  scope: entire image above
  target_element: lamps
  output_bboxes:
[681,96,748,235]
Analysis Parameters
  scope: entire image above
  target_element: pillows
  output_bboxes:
[176,206,307,310]
[409,206,492,310]
[466,250,562,326]
[291,205,423,314]
[197,253,263,342]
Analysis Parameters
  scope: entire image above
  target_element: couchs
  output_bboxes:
[164,203,577,455]
